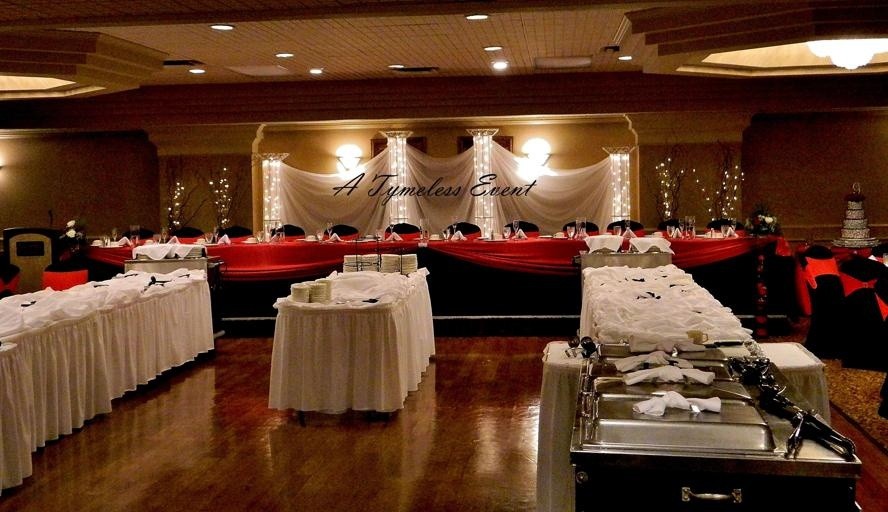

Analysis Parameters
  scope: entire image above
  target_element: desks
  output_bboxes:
[86,232,792,337]
[267,265,437,412]
[1,268,220,492]
[536,263,867,511]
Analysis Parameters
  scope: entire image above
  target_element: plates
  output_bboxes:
[538,235,554,238]
[342,253,418,274]
[303,239,318,243]
[241,240,257,244]
[138,246,203,260]
[291,279,333,304]
[555,236,568,240]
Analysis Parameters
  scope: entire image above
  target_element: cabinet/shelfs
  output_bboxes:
[569,248,674,270]
[123,256,228,339]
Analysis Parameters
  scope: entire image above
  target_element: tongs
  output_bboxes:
[786,414,857,463]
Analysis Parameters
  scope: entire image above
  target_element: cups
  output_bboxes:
[257,231,265,243]
[503,227,511,239]
[613,226,622,237]
[686,330,709,345]
[567,226,575,238]
[375,229,385,243]
[442,229,450,240]
[306,235,316,241]
[553,232,565,238]
[653,232,663,238]
[666,226,675,237]
[247,238,257,243]
[430,234,439,240]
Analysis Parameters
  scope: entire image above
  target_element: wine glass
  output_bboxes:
[389,216,394,234]
[112,227,219,243]
[451,215,458,234]
[326,221,333,239]
[316,228,325,245]
[679,219,685,237]
[513,218,520,237]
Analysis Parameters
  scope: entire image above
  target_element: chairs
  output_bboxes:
[796,246,888,373]
